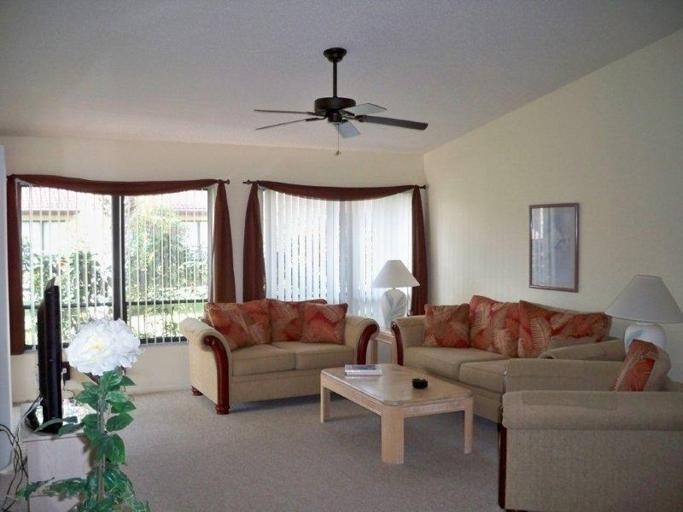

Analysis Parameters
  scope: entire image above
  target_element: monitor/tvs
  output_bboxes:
[27,285,88,431]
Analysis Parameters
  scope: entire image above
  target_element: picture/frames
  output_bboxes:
[528,202,580,293]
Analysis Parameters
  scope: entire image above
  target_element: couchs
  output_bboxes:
[390,295,623,423]
[180,298,379,414]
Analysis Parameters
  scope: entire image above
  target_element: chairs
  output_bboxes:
[498,339,682,512]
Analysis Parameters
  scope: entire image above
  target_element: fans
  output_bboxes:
[253,47,428,156]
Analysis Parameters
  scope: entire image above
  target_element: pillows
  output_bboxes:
[299,302,350,344]
[423,304,473,348]
[202,302,257,354]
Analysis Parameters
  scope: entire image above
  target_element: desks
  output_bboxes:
[377,330,394,363]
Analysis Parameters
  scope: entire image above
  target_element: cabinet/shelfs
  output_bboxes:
[19,400,95,512]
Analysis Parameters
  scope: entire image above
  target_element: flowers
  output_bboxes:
[16,319,151,512]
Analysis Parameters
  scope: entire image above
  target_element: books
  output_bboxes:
[344,364,376,373]
[347,366,382,375]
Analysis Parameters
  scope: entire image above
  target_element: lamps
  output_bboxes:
[368,260,420,330]
[603,274,682,355]
